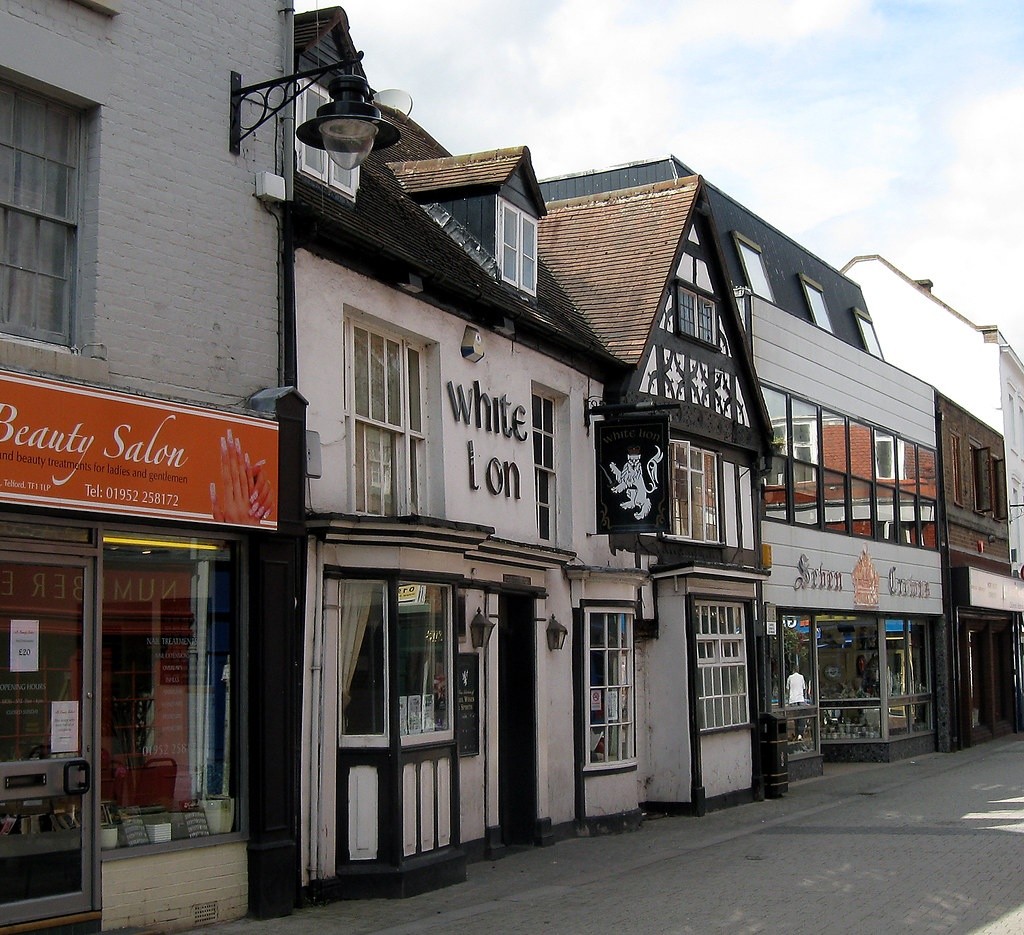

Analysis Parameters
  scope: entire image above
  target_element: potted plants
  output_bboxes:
[101,824,118,849]
[140,812,171,843]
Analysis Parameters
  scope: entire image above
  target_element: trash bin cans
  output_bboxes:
[760,712,788,798]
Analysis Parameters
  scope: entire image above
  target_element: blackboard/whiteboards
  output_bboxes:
[118,806,152,847]
[180,799,209,838]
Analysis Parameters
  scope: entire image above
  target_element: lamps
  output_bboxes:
[546,614,568,652]
[227,52,401,171]
[989,534,998,542]
[471,606,496,649]
